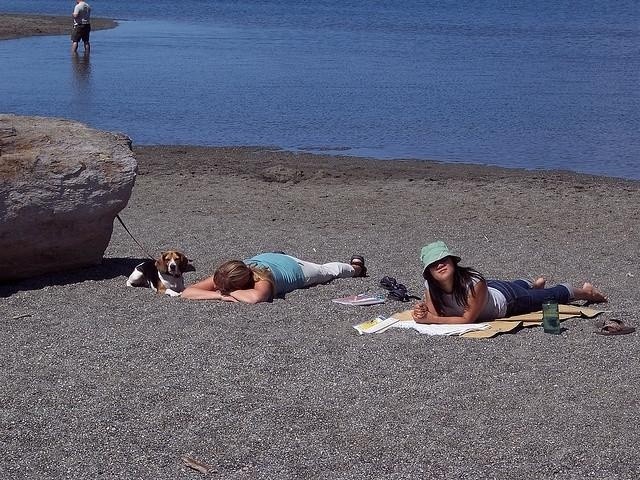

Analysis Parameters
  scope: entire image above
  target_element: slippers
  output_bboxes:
[595,319,636,336]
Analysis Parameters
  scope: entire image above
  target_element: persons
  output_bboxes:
[180,251,368,304]
[71,0,91,57]
[413,240,607,324]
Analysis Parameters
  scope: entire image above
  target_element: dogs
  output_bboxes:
[127,251,188,297]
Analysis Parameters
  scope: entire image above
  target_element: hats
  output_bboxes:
[419,239,461,281]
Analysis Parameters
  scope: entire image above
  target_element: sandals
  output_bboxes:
[348,254,369,277]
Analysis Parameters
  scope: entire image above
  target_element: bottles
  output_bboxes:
[541,294,561,335]
